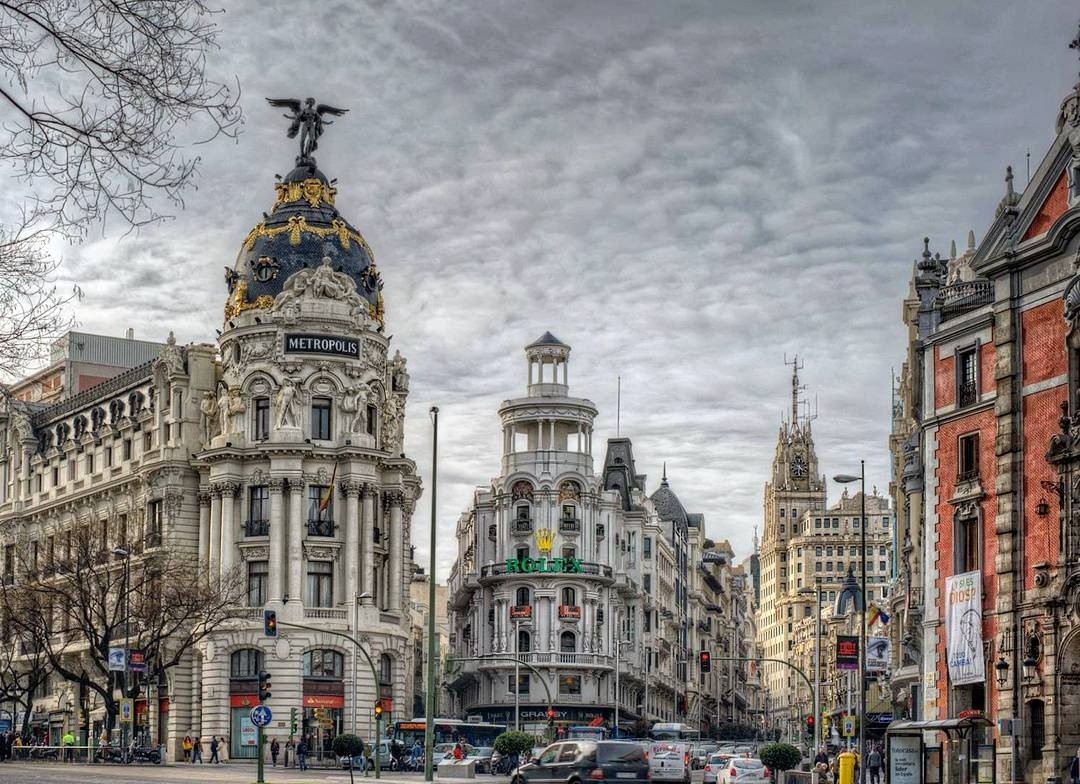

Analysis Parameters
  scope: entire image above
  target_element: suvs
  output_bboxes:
[509,738,653,784]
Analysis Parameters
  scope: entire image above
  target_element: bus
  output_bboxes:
[395,718,509,766]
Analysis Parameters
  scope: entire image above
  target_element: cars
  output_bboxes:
[430,743,473,767]
[715,758,772,783]
[648,737,760,784]
[341,738,405,771]
[471,747,547,783]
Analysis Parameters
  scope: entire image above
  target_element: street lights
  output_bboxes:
[112,548,131,764]
[832,459,867,783]
[645,649,658,715]
[424,405,439,782]
[352,591,374,738]
[718,676,728,727]
[515,621,533,731]
[798,584,823,761]
[615,639,632,737]
[674,659,687,722]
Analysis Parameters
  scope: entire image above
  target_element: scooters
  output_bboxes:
[93,736,161,764]
[30,744,59,762]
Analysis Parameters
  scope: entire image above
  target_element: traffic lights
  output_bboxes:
[805,716,815,734]
[373,700,382,720]
[257,670,272,700]
[264,609,278,639]
[699,651,710,673]
[291,724,297,733]
[290,708,299,723]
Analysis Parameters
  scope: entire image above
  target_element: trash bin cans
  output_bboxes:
[838,753,855,784]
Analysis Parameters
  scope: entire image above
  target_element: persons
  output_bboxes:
[182,735,228,765]
[270,737,307,771]
[389,738,467,772]
[814,744,886,784]
[0,730,37,762]
[63,731,74,763]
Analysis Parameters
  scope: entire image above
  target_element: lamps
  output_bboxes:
[1035,493,1048,517]
[994,628,1012,688]
[1022,632,1039,685]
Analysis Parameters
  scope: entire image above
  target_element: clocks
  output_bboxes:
[791,458,808,476]
